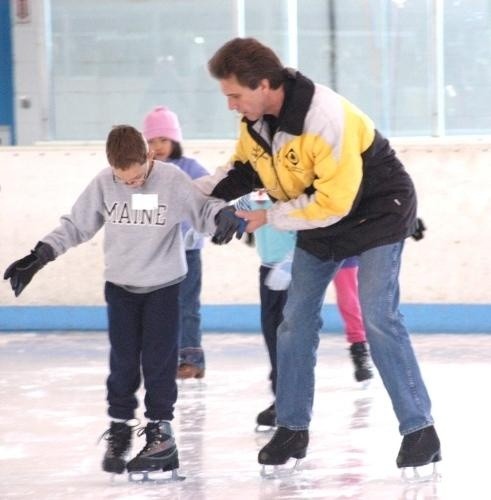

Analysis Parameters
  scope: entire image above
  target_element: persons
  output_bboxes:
[3,125,248,474]
[331,256,373,382]
[232,188,298,427]
[144,107,212,378]
[191,38,441,469]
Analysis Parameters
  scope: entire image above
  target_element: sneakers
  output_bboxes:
[175,363,205,380]
[345,340,374,381]
[257,402,278,427]
[258,426,310,465]
[125,420,180,473]
[95,416,141,475]
[396,424,442,468]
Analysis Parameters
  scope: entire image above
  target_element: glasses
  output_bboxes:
[111,162,147,184]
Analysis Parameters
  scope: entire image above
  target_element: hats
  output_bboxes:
[143,105,182,145]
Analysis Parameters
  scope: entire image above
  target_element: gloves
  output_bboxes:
[3,241,56,298]
[211,204,247,245]
